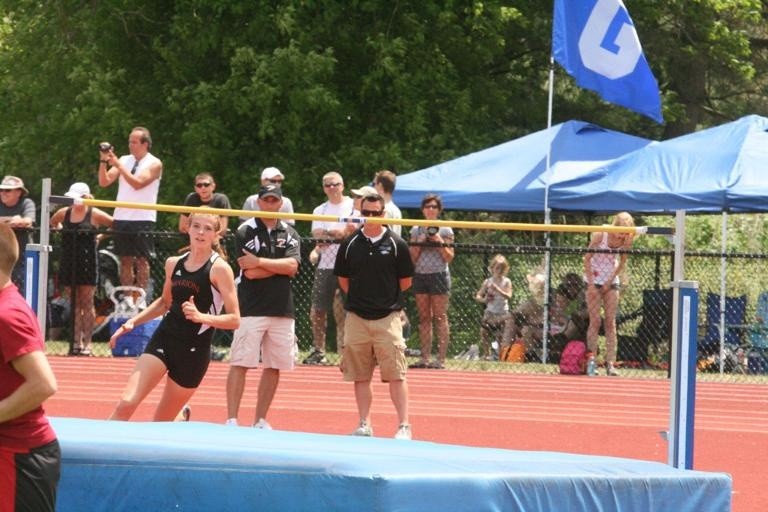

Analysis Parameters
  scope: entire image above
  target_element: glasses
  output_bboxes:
[195,181,212,189]
[423,203,439,211]
[323,181,342,190]
[359,207,384,218]
[263,178,282,184]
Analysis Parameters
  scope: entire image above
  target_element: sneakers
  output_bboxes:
[120,296,134,311]
[302,347,327,367]
[588,358,620,378]
[135,296,148,312]
[353,417,373,436]
[393,422,413,440]
[71,342,92,355]
[211,344,227,362]
[181,404,192,422]
[224,417,239,427]
[254,417,273,430]
[407,355,444,371]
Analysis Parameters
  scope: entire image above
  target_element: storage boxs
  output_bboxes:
[112,307,163,360]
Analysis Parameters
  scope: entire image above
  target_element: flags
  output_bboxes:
[549,0,664,124]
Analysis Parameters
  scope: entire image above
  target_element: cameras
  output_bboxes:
[427,226,439,235]
[100,144,111,152]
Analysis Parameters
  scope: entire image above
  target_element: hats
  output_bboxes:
[257,183,282,201]
[349,184,378,197]
[260,166,285,181]
[63,182,94,201]
[0,175,29,194]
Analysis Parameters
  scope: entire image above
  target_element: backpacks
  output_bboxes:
[559,340,591,376]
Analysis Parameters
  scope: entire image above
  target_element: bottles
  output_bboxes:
[586,351,596,375]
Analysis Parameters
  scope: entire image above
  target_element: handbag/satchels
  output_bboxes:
[501,343,528,364]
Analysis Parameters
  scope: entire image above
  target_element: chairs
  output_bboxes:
[738,290,768,372]
[619,288,674,363]
[701,292,747,371]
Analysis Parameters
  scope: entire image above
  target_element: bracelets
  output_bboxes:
[99,158,108,164]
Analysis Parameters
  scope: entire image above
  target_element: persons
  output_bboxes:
[0,223,62,512]
[107,168,455,441]
[474,209,637,378]
[1,176,36,295]
[98,126,164,310]
[51,183,113,356]
